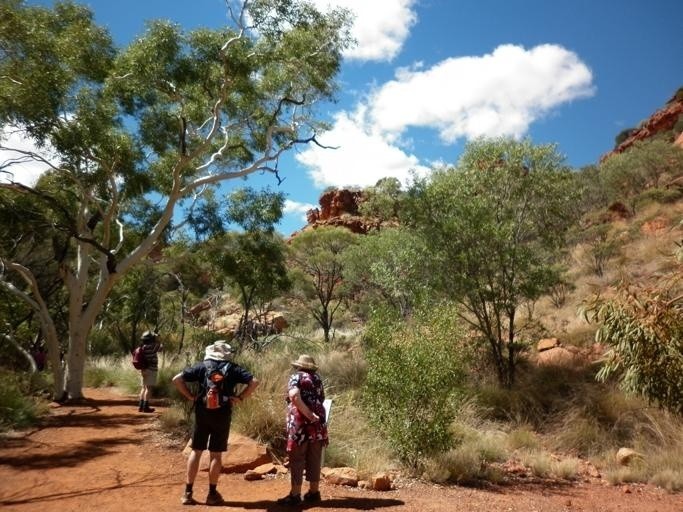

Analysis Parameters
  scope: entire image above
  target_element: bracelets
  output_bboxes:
[310,413,315,421]
[238,395,243,401]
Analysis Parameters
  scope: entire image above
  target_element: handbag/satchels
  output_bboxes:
[131,345,144,370]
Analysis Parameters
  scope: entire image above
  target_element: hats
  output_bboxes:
[290,354,319,371]
[139,330,158,340]
[203,340,232,361]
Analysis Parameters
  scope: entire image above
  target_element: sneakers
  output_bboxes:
[277,489,320,509]
[179,491,193,504]
[138,407,154,412]
[205,490,223,505]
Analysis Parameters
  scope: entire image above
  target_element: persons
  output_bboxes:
[138,330,161,412]
[172,339,260,505]
[278,354,330,505]
[33,346,48,371]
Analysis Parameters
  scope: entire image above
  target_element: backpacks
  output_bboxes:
[194,360,233,424]
